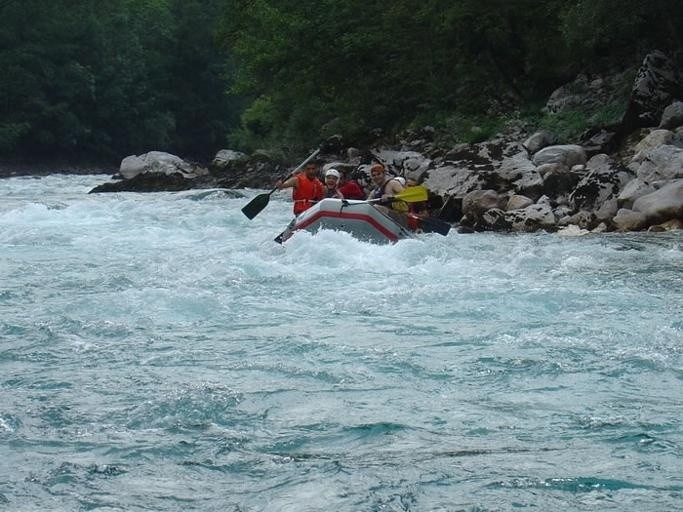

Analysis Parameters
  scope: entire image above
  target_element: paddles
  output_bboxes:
[241,149,320,221]
[405,213,451,236]
[348,186,428,205]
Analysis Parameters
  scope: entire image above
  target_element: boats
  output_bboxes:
[280,197,414,246]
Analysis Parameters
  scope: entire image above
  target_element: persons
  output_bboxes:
[275,159,444,234]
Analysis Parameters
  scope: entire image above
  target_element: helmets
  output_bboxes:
[325,169,340,189]
[394,177,406,185]
